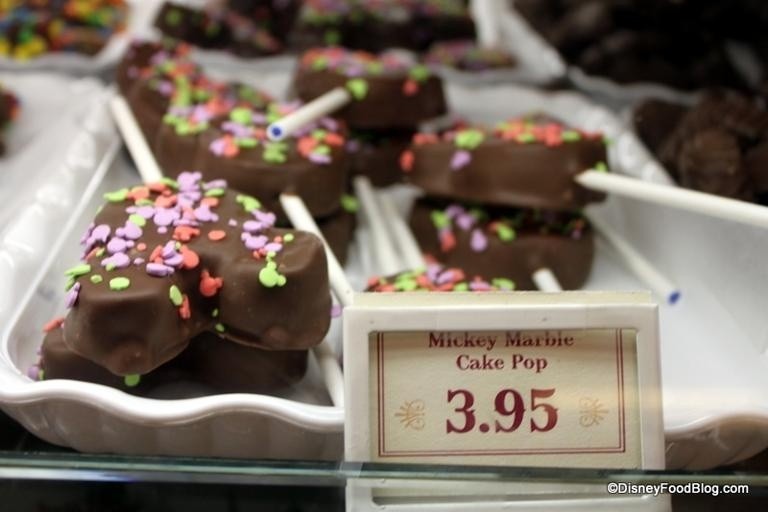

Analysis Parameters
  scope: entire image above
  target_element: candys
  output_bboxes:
[0,0,767,395]
